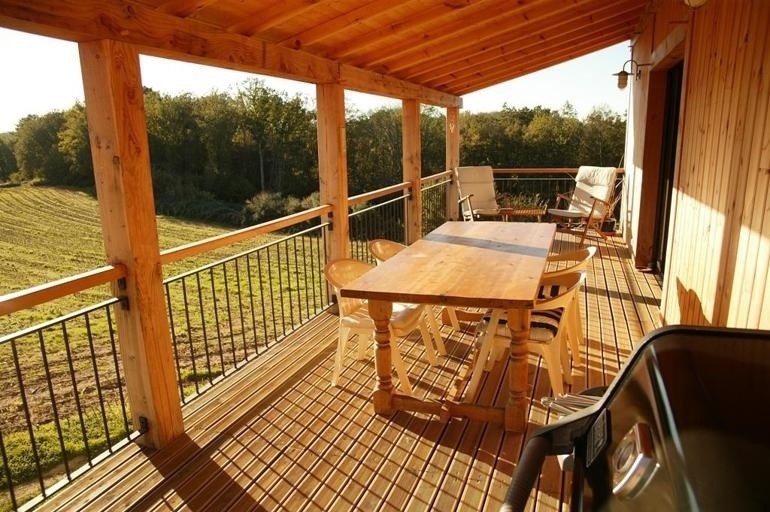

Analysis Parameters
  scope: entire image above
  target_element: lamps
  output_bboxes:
[613,59,652,90]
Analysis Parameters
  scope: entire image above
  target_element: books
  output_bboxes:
[538,392,603,416]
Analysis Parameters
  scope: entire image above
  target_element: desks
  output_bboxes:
[340,221,555,431]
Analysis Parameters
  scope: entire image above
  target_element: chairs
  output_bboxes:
[369,239,462,354]
[451,165,511,221]
[547,166,615,247]
[546,248,598,346]
[326,258,440,393]
[462,270,585,403]
